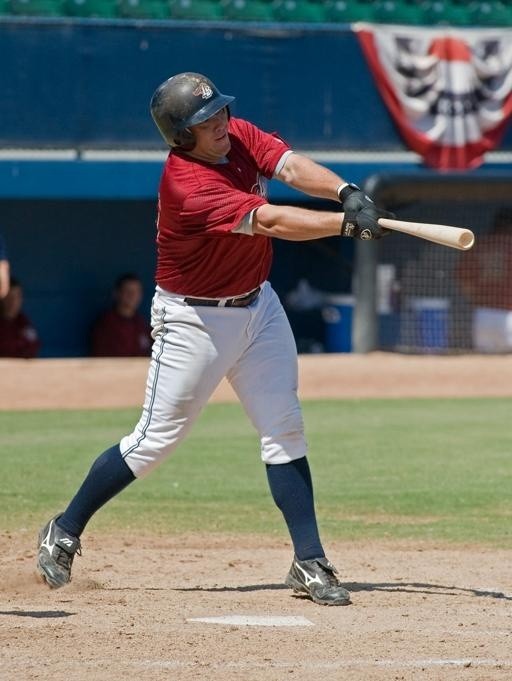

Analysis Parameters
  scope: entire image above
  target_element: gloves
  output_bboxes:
[338,183,397,241]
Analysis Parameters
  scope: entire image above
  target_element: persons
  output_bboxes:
[72,275,152,356]
[34,70,381,608]
[1,279,43,359]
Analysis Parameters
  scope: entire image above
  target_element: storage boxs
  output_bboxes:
[394,297,449,349]
[324,295,399,351]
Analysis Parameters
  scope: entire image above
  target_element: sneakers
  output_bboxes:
[285,555,350,605]
[37,512,82,587]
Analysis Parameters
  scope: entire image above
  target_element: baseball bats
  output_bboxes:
[377,217,475,252]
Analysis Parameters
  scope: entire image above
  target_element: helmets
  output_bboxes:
[150,72,236,152]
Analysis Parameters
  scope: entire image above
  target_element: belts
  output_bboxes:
[184,287,261,307]
